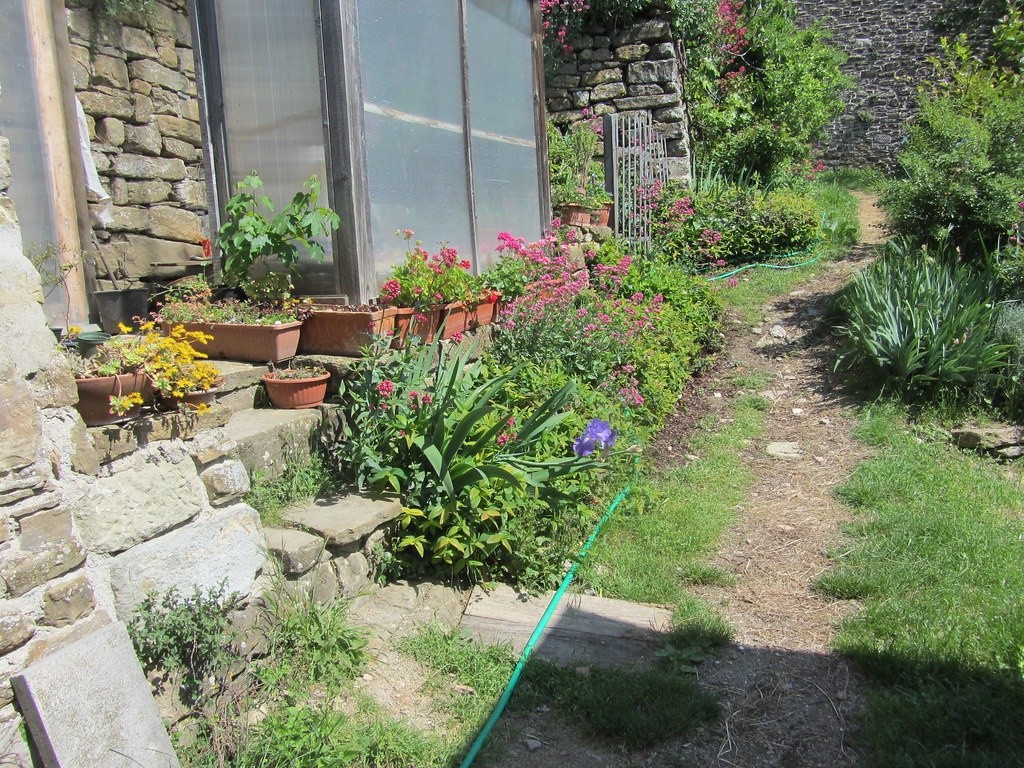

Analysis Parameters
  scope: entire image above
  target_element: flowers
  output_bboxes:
[267,367,326,380]
[59,284,314,432]
[379,230,572,309]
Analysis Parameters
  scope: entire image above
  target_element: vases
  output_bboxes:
[262,366,331,409]
[93,287,151,334]
[76,331,112,360]
[159,319,303,364]
[390,304,442,350]
[464,294,496,330]
[160,387,218,412]
[438,300,466,340]
[524,282,539,306]
[293,306,398,359]
[491,292,506,324]
[72,374,144,428]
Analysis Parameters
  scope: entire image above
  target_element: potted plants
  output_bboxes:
[560,181,590,228]
[594,186,613,226]
[585,194,602,227]
